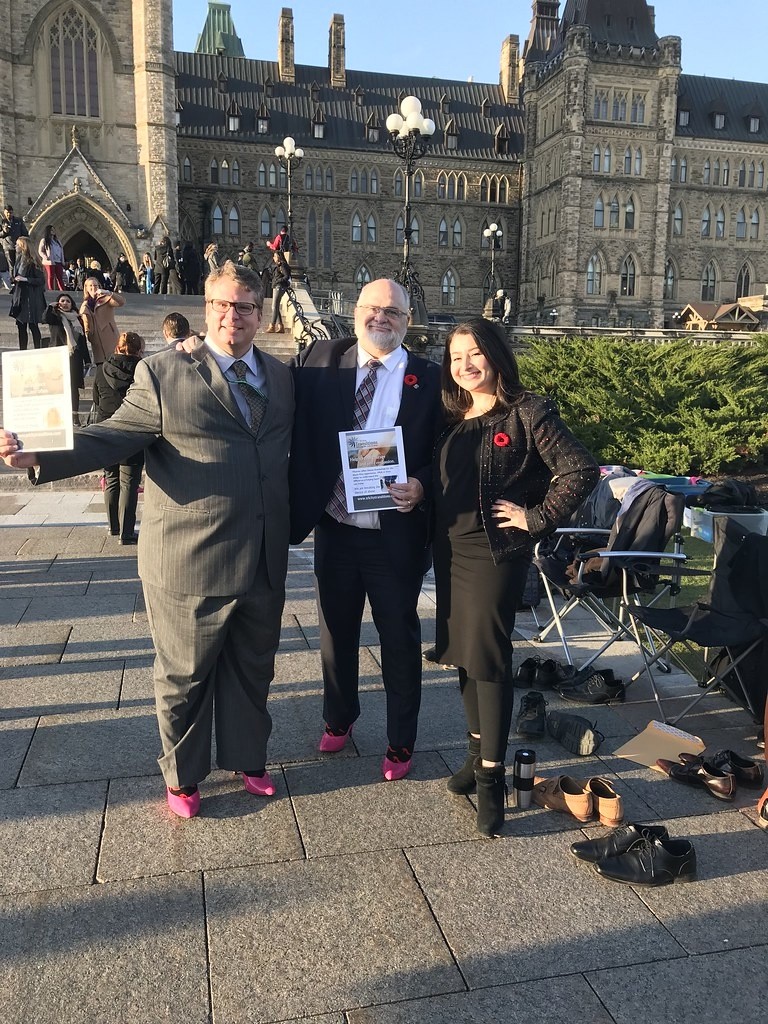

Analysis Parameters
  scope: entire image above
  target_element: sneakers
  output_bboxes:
[532,774,594,823]
[512,655,626,756]
[574,776,624,826]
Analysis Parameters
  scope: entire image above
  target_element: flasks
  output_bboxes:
[513,748,536,810]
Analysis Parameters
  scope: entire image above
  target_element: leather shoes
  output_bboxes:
[568,822,669,862]
[687,749,764,790]
[657,759,677,772]
[678,753,697,765]
[669,762,737,802]
[594,827,696,886]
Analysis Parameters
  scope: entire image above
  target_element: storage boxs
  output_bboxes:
[691,504,768,542]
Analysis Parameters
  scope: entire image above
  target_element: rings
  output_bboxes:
[407,500,411,508]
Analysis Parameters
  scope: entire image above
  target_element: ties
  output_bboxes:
[232,360,266,434]
[329,360,381,524]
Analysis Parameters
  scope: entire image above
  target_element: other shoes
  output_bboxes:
[107,527,119,536]
[118,532,138,545]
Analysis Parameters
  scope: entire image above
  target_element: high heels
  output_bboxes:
[233,768,275,796]
[383,742,413,780]
[165,785,200,818]
[319,718,354,752]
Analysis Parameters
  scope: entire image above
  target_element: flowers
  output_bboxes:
[493,433,510,448]
[404,374,418,385]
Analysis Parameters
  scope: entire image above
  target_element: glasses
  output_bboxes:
[356,305,409,319]
[204,298,258,315]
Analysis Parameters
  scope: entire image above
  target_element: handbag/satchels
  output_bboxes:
[162,246,175,270]
[9,301,23,318]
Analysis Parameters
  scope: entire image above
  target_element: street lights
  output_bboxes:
[386,97,437,321]
[274,137,305,251]
[483,223,504,317]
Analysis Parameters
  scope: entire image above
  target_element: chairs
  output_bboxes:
[532,474,768,744]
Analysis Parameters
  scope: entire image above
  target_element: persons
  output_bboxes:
[175,278,453,780]
[0,261,294,820]
[0,204,301,545]
[433,317,599,833]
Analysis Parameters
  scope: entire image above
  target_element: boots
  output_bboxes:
[262,323,274,333]
[472,755,505,834]
[446,730,482,795]
[276,324,285,333]
